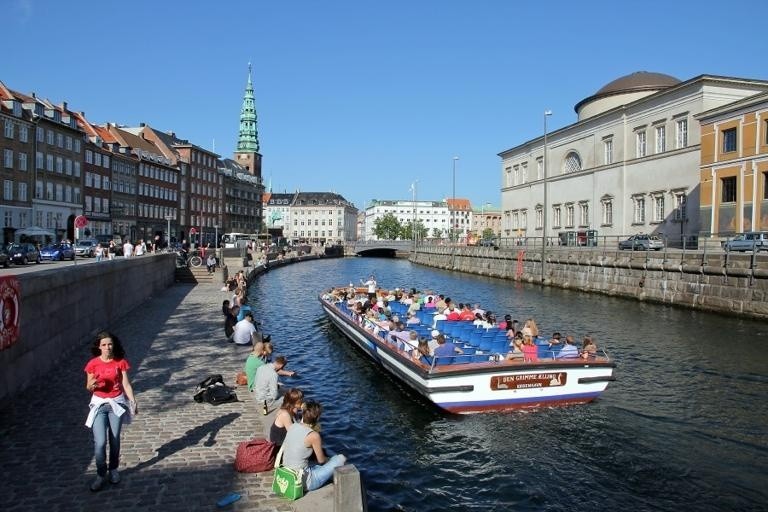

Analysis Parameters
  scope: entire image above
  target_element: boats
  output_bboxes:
[318,282,616,416]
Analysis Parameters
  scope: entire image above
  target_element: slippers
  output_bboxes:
[216,493,241,506]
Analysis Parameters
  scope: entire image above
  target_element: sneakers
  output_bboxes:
[109,468,120,483]
[91,475,105,492]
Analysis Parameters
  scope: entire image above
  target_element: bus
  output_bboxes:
[220,233,258,249]
[255,233,272,246]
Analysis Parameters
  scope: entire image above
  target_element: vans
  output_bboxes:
[719,231,767,252]
[94,234,122,253]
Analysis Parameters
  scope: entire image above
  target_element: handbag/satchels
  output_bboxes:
[271,463,304,501]
[234,437,276,473]
[236,371,248,385]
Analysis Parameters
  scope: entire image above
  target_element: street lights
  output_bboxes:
[409,178,423,239]
[213,225,219,249]
[452,156,460,242]
[541,109,553,282]
[164,215,174,247]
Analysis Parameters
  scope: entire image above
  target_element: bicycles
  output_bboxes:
[174,249,203,269]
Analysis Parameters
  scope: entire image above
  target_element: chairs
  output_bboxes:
[335,296,564,367]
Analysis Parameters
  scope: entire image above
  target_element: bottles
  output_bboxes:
[264,399,268,415]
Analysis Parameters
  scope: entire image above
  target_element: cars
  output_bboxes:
[8,243,41,264]
[618,233,663,251]
[38,243,76,261]
[75,240,98,258]
[0,243,12,267]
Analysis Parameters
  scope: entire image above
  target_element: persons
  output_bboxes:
[270,388,306,448]
[84,331,137,492]
[94,243,105,260]
[253,356,288,410]
[277,252,284,263]
[122,237,190,268]
[328,274,598,364]
[107,240,117,260]
[222,238,271,346]
[279,399,348,493]
[206,254,217,275]
[243,342,272,392]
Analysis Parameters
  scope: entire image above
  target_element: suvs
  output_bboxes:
[480,237,497,246]
